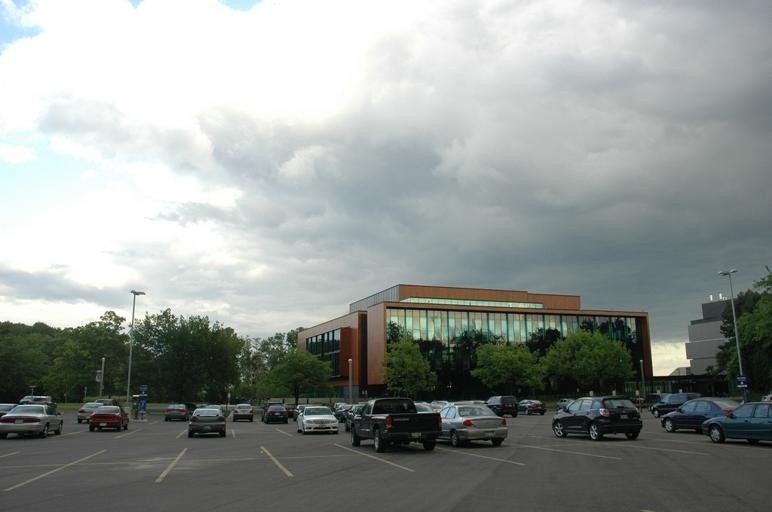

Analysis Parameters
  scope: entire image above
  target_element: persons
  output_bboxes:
[743,388,750,402]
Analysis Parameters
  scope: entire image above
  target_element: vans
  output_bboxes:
[552,395,642,440]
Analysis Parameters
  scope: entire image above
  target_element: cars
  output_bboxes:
[78,397,129,432]
[0,394,63,438]
[261,395,547,452]
[232,403,254,421]
[650,392,772,442]
[165,403,226,437]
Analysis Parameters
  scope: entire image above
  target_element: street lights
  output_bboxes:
[126,290,146,406]
[99,357,105,398]
[348,358,352,404]
[718,269,743,384]
